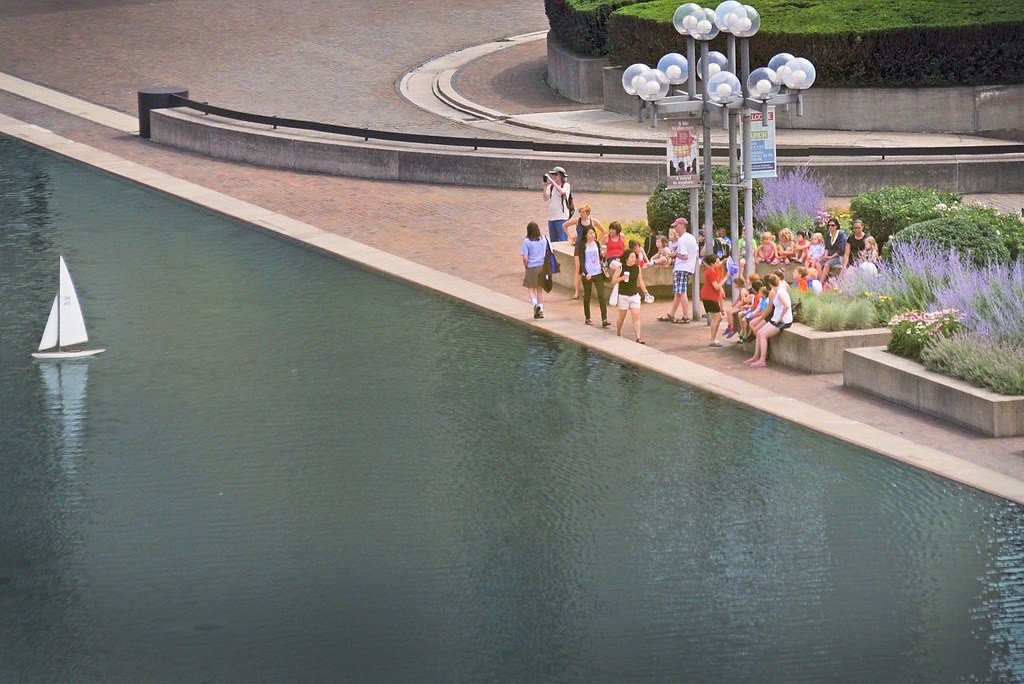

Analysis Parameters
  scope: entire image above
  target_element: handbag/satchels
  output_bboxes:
[549,254,561,274]
[542,253,552,293]
[602,266,611,282]
[609,265,622,306]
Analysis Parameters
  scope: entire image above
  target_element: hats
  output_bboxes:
[549,167,566,177]
[671,218,688,226]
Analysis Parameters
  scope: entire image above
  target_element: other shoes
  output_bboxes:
[586,318,592,325]
[534,305,545,319]
[749,359,766,367]
[702,314,708,318]
[783,257,790,265]
[742,358,757,364]
[709,342,722,348]
[771,258,781,265]
[791,257,802,264]
[603,320,611,327]
[724,326,755,343]
[573,293,579,299]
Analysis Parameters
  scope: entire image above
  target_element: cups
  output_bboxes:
[601,244,607,254]
[624,272,630,282]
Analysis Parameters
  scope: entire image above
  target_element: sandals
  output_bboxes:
[637,339,645,344]
[674,317,690,325]
[656,313,674,322]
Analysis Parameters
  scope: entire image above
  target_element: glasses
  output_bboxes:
[828,224,837,227]
[552,173,560,176]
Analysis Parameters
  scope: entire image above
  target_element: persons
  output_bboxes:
[792,231,810,263]
[722,266,844,344]
[543,167,573,242]
[601,221,758,267]
[700,255,731,345]
[656,218,698,323]
[670,158,696,176]
[520,222,560,318]
[806,232,826,272]
[840,220,873,278]
[744,276,794,367]
[850,254,883,281]
[562,204,606,299]
[814,219,848,282]
[755,232,776,264]
[578,227,612,326]
[771,228,794,265]
[612,250,648,344]
[862,236,882,265]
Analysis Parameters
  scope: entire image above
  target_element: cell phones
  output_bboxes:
[543,175,547,182]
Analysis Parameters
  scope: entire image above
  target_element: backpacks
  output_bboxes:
[551,184,575,219]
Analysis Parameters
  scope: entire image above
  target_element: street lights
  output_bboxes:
[621,0,816,322]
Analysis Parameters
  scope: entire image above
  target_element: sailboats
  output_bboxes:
[31,254,106,359]
